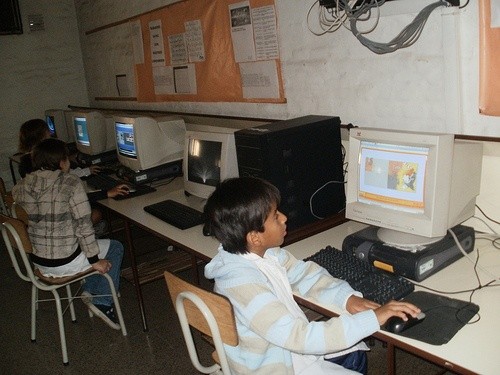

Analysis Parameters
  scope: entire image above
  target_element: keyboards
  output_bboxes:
[86,174,120,192]
[144,199,208,230]
[302,246,417,306]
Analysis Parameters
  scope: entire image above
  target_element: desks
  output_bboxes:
[8,150,500,375]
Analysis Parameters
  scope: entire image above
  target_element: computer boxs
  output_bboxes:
[343,226,476,283]
[115,161,180,184]
[234,115,349,247]
[77,150,118,166]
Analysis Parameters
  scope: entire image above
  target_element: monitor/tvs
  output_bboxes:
[345,128,484,247]
[45,107,239,201]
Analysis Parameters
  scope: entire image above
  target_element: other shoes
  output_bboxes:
[93,220,108,234]
[81,292,121,330]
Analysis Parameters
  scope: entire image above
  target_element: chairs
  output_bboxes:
[5,191,29,229]
[164,269,239,375]
[0,215,127,366]
[0,177,9,217]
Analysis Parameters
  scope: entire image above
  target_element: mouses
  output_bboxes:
[123,186,137,193]
[203,225,215,236]
[384,313,426,335]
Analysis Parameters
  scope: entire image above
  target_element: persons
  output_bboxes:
[18,119,101,178]
[12,138,124,318]
[204,177,425,375]
